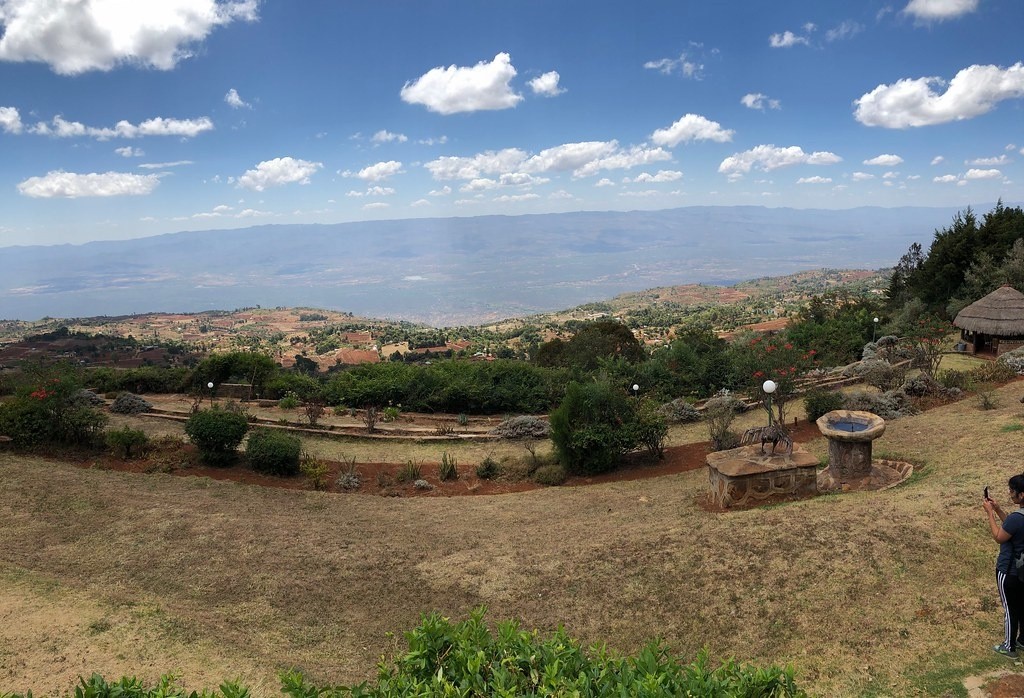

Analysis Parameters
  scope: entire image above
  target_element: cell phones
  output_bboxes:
[984,486,988,498]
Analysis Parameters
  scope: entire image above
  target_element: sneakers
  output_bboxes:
[1015,642,1024,650]
[993,645,1018,659]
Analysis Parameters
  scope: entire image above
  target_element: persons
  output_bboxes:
[983,474,1024,660]
